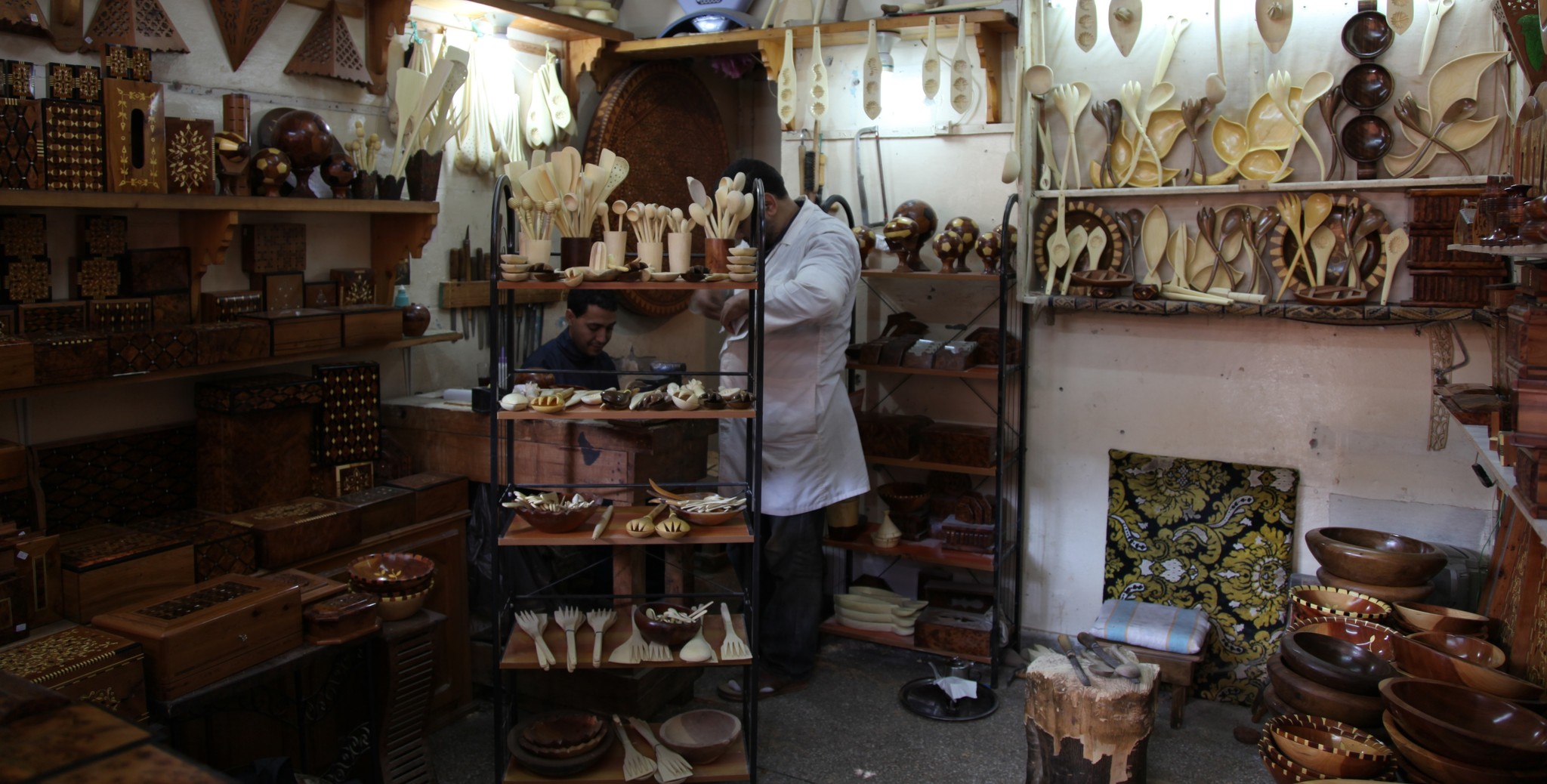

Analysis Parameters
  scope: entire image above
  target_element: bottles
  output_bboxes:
[395,284,410,306]
[402,302,431,337]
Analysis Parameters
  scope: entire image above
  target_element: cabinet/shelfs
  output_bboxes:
[490,174,760,784]
[816,194,1036,691]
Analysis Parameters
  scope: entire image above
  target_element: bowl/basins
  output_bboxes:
[634,602,701,645]
[660,708,742,765]
[507,708,616,778]
[1254,525,1547,783]
[347,552,436,622]
[511,491,602,534]
[670,492,744,525]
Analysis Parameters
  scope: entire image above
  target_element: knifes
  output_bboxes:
[1059,632,1141,686]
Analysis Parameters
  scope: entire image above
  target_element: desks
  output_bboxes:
[379,385,719,615]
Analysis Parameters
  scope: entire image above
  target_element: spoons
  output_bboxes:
[635,756,657,780]
[499,380,757,412]
[501,171,758,281]
[645,601,713,624]
[626,504,689,539]
[678,496,747,512]
[389,40,526,182]
[655,769,687,784]
[999,11,1509,310]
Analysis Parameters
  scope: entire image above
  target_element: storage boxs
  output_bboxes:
[0,55,474,721]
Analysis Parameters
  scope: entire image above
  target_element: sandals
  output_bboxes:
[719,671,813,700]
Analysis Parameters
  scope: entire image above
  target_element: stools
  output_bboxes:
[1081,633,1211,732]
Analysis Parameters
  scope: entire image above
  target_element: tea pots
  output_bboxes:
[926,657,981,686]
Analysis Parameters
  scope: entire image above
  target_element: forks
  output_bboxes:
[515,610,556,665]
[502,491,595,511]
[558,606,579,665]
[613,714,648,776]
[721,601,747,657]
[591,608,612,662]
[629,605,650,661]
[628,716,692,773]
[649,640,670,659]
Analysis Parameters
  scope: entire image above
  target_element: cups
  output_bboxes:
[402,149,443,200]
[354,169,379,198]
[377,174,405,200]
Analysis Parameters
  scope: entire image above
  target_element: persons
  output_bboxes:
[687,159,871,705]
[517,289,621,390]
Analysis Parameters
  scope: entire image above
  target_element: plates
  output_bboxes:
[898,678,999,721]
[826,482,937,636]
